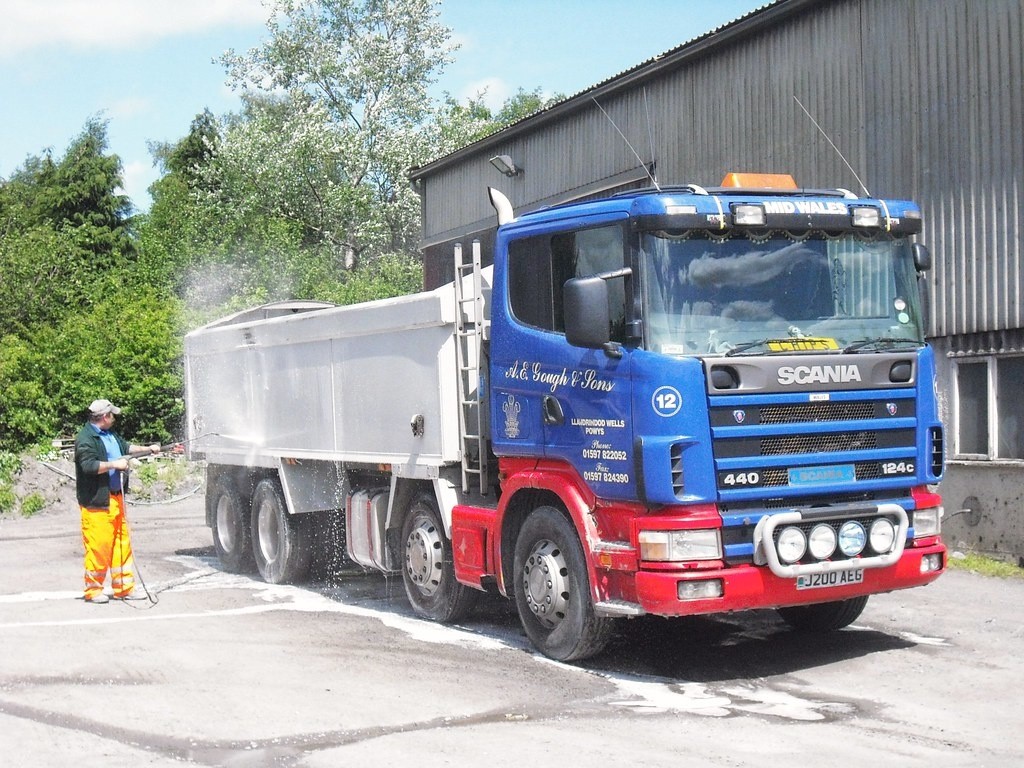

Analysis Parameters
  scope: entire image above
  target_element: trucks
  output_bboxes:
[183,171,950,662]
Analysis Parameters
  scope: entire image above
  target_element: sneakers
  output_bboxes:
[85,587,110,604]
[113,589,149,599]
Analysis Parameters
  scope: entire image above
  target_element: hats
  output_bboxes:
[88,399,121,416]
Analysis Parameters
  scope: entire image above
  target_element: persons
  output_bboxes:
[74,398,160,601]
